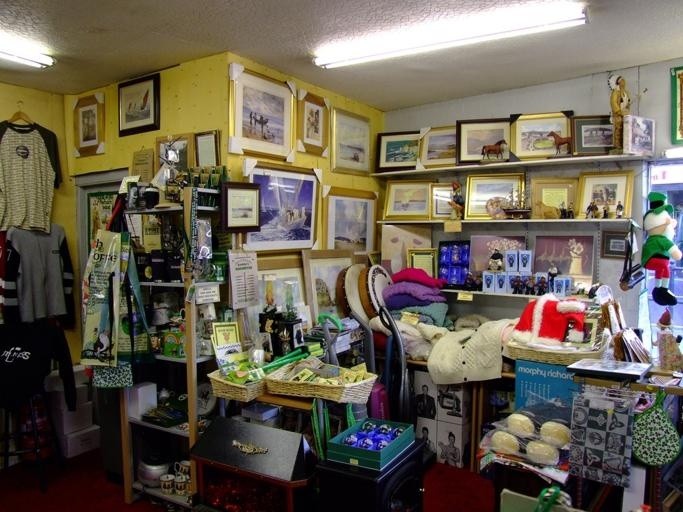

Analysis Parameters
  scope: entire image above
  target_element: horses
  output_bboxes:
[482,140,508,160]
[547,130,572,157]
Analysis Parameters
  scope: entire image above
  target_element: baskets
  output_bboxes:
[206,363,266,402]
[507,333,610,365]
[267,359,376,405]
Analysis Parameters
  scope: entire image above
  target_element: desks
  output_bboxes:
[475,371,516,474]
[406,359,476,471]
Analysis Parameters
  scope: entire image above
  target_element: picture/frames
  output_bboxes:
[373,128,420,172]
[227,62,296,161]
[72,71,220,170]
[600,231,628,259]
[328,104,372,177]
[427,182,462,219]
[294,87,330,159]
[217,156,380,331]
[414,124,455,169]
[381,178,438,221]
[450,109,636,221]
[404,248,438,281]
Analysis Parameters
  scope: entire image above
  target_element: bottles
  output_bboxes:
[144,183,159,210]
[618,264,644,291]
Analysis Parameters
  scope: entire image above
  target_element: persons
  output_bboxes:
[419,428,435,452]
[609,73,631,151]
[438,432,460,466]
[416,383,435,419]
[486,198,625,296]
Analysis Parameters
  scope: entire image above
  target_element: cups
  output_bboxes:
[174,459,190,474]
[174,475,189,495]
[160,474,174,494]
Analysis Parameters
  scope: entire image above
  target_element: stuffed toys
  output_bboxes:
[642,192,681,304]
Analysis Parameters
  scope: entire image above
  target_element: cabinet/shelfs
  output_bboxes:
[366,152,641,225]
[112,177,218,507]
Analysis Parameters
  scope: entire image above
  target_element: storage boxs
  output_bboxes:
[327,417,414,472]
[413,371,470,469]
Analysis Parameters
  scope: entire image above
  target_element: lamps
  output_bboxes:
[311,5,587,69]
[0,46,57,71]
